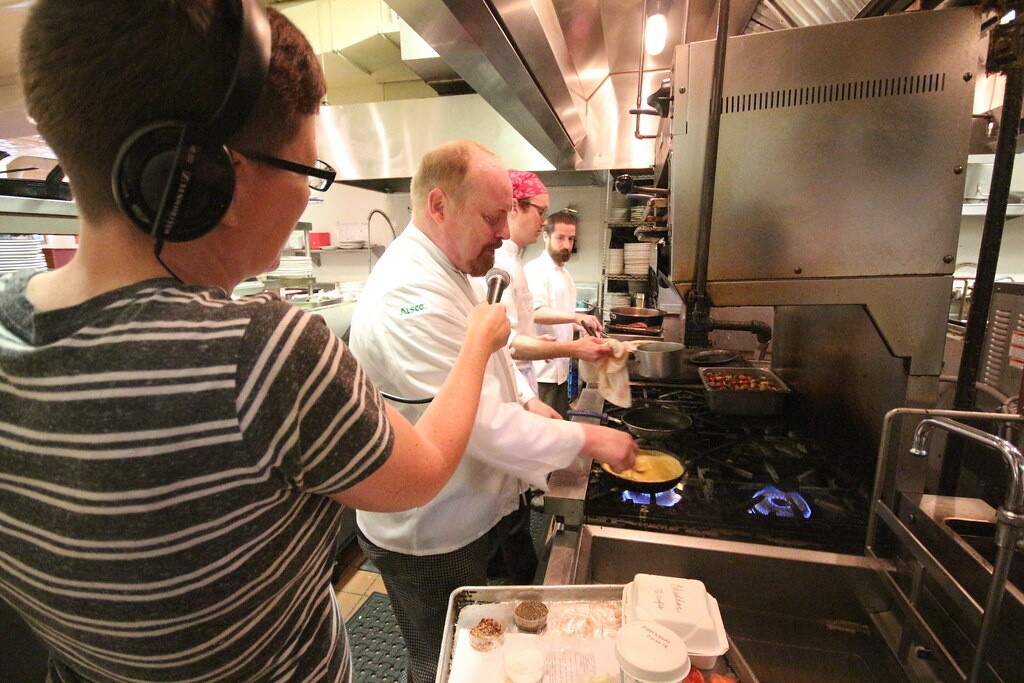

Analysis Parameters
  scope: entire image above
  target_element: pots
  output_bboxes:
[599,444,686,494]
[582,300,668,326]
[566,407,692,441]
[963,162,993,200]
[627,341,685,379]
[0,162,76,201]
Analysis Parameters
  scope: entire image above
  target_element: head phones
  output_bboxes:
[112,0,271,243]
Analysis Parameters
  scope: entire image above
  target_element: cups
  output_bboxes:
[613,622,691,683]
[510,591,552,630]
[503,633,546,682]
[310,232,330,249]
[467,610,509,651]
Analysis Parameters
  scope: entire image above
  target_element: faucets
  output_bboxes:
[908,415,1024,682]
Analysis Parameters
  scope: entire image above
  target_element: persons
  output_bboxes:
[349,139,640,683]
[467,170,612,399]
[523,212,603,420]
[0,0,513,683]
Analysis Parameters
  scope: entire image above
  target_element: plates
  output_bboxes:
[691,349,739,364]
[232,281,265,296]
[267,256,312,277]
[318,240,365,250]
[603,293,631,321]
[607,242,653,276]
[0,239,48,276]
[609,205,646,222]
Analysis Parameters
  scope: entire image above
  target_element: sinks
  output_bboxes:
[584,534,976,683]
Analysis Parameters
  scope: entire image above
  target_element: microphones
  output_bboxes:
[484,268,510,305]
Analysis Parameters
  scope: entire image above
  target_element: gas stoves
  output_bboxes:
[581,384,885,540]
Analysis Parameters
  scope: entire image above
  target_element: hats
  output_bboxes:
[506,168,548,200]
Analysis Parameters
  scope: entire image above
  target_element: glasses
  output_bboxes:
[231,145,336,192]
[517,198,551,220]
